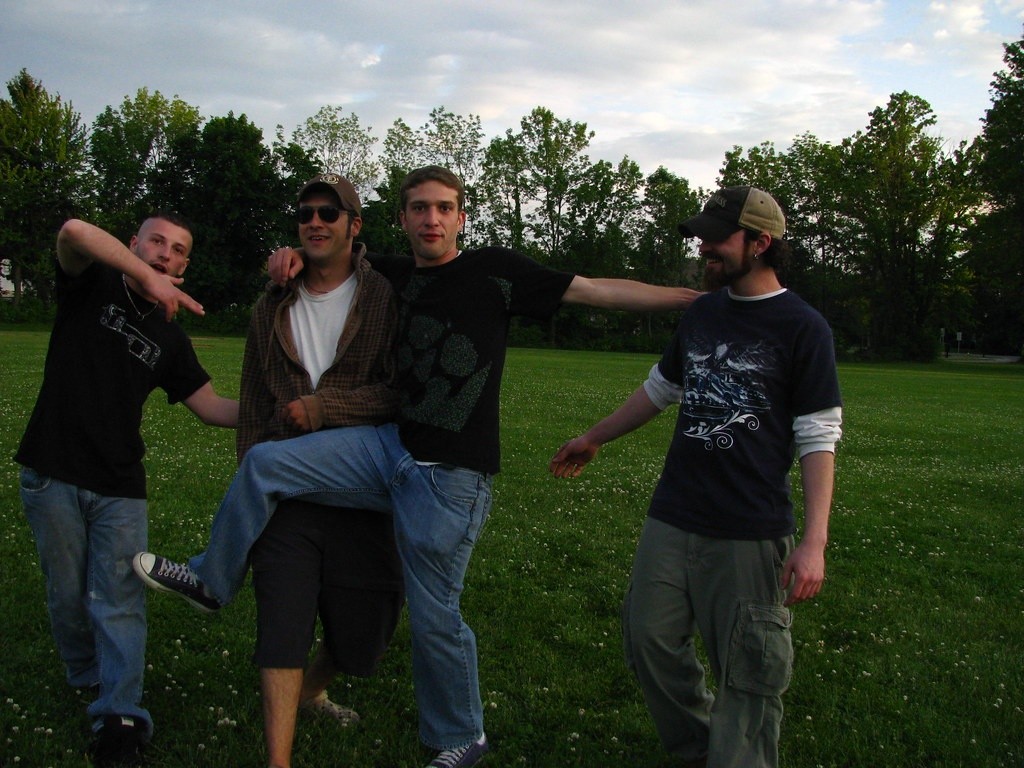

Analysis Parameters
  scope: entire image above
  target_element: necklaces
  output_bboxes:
[306,282,335,294]
[123,272,159,322]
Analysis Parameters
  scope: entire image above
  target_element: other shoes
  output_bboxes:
[84,715,148,768]
[64,680,100,698]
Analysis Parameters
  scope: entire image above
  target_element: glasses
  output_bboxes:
[296,206,355,224]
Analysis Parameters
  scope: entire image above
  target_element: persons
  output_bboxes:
[13,211,240,768]
[132,165,710,768]
[549,185,843,768]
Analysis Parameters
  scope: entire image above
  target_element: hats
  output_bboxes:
[296,173,361,218]
[677,187,785,240]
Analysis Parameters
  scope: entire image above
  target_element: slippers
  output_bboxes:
[298,690,360,726]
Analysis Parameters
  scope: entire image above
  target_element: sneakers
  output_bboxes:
[425,732,489,768]
[132,552,221,613]
